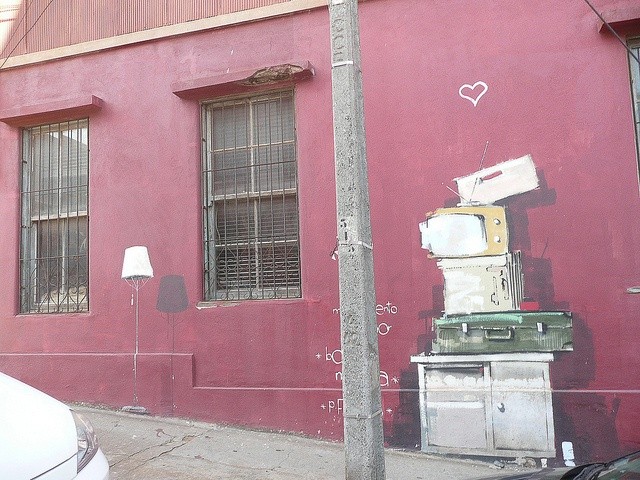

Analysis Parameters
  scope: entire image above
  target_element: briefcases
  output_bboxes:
[430,311,574,355]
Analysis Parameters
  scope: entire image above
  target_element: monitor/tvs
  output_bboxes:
[418,205,510,260]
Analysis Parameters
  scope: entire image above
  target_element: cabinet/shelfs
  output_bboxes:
[411,353,556,468]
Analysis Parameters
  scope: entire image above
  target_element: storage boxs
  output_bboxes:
[454,153,538,206]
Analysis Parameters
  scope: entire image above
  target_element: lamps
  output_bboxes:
[119,246,156,415]
[158,275,190,418]
